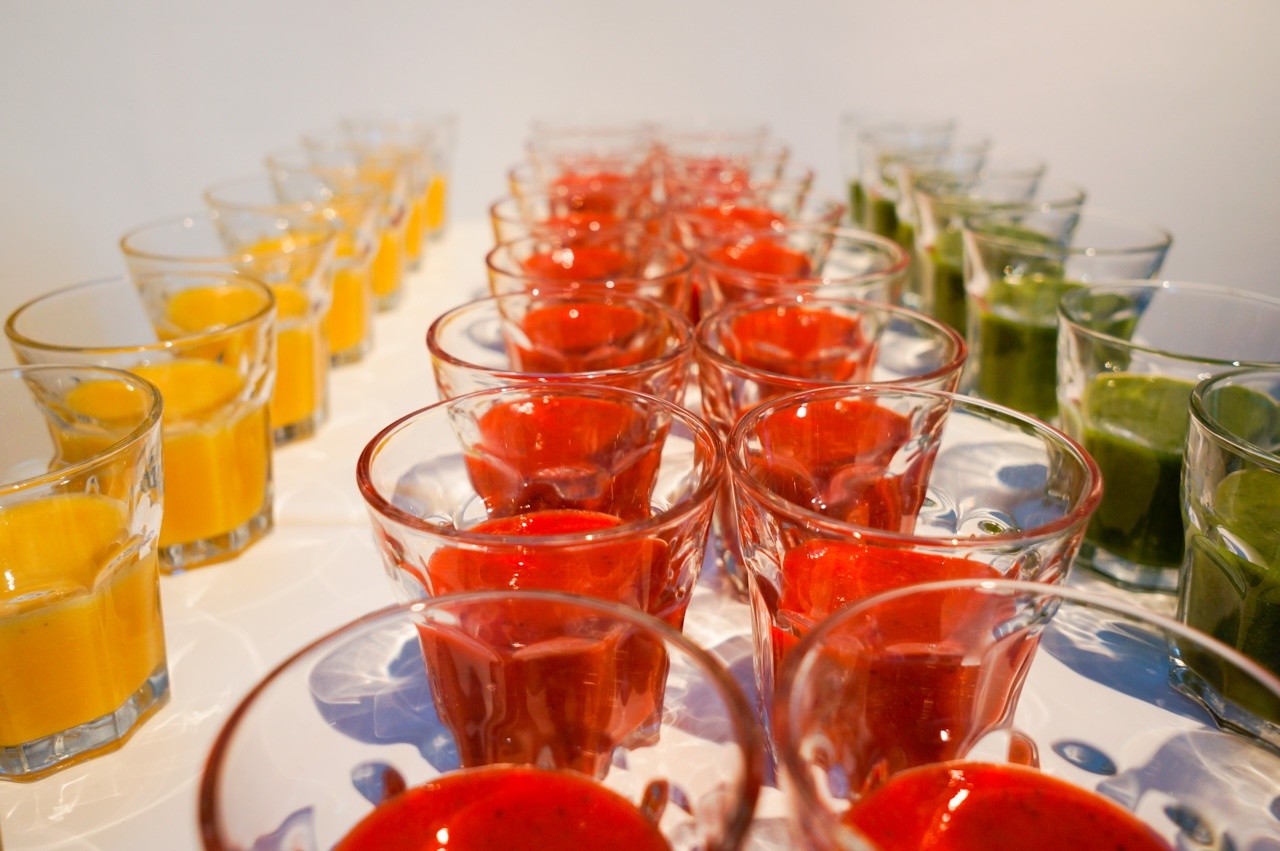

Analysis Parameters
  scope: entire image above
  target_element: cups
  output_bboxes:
[6,271,277,576]
[661,130,814,210]
[485,228,693,372]
[200,590,762,851]
[667,189,847,275]
[697,299,968,604]
[508,120,663,221]
[203,120,446,368]
[846,126,994,294]
[121,211,336,446]
[771,579,1280,851]
[490,192,666,237]
[907,174,1084,341]
[428,290,697,522]
[730,384,1105,788]
[356,383,728,779]
[953,206,1171,432]
[1053,280,1280,595]
[1,366,171,783]
[1168,366,1280,754]
[698,223,909,306]
